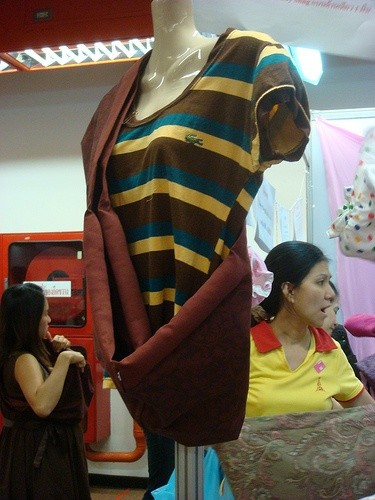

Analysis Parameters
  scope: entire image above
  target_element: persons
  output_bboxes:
[0,283,91,500]
[81,0,310,449]
[217,240,375,419]
